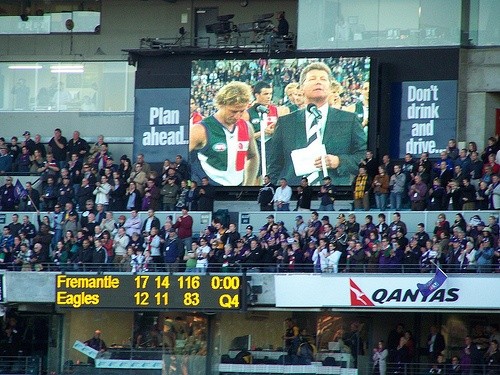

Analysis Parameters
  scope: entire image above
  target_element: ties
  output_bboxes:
[308,111,323,186]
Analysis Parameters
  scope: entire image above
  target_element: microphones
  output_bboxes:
[253,104,270,114]
[306,103,322,119]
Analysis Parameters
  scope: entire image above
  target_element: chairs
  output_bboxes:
[322,356,337,366]
[221,354,233,364]
[276,355,293,364]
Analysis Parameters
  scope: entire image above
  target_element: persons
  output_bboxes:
[84,330,109,367]
[3,313,24,356]
[281,318,307,361]
[269,11,290,55]
[135,328,160,347]
[0,128,500,274]
[190,57,370,186]
[334,320,500,375]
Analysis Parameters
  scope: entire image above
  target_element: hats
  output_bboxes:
[482,227,491,233]
[107,157,112,160]
[168,177,174,180]
[322,176,331,181]
[337,214,345,219]
[277,220,284,225]
[295,216,302,221]
[321,216,329,220]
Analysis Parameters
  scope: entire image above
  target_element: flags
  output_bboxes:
[417,268,448,296]
